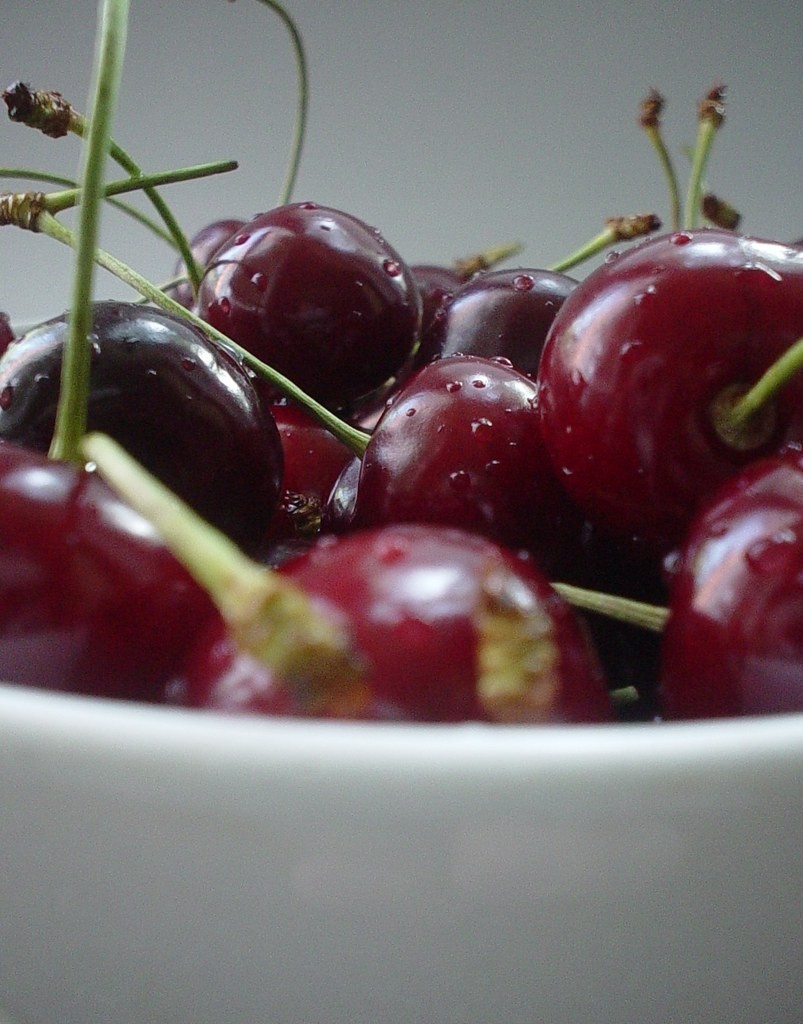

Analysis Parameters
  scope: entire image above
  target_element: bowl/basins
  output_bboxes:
[1,681,803,1024]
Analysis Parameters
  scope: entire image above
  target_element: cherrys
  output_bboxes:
[0,82,803,727]
